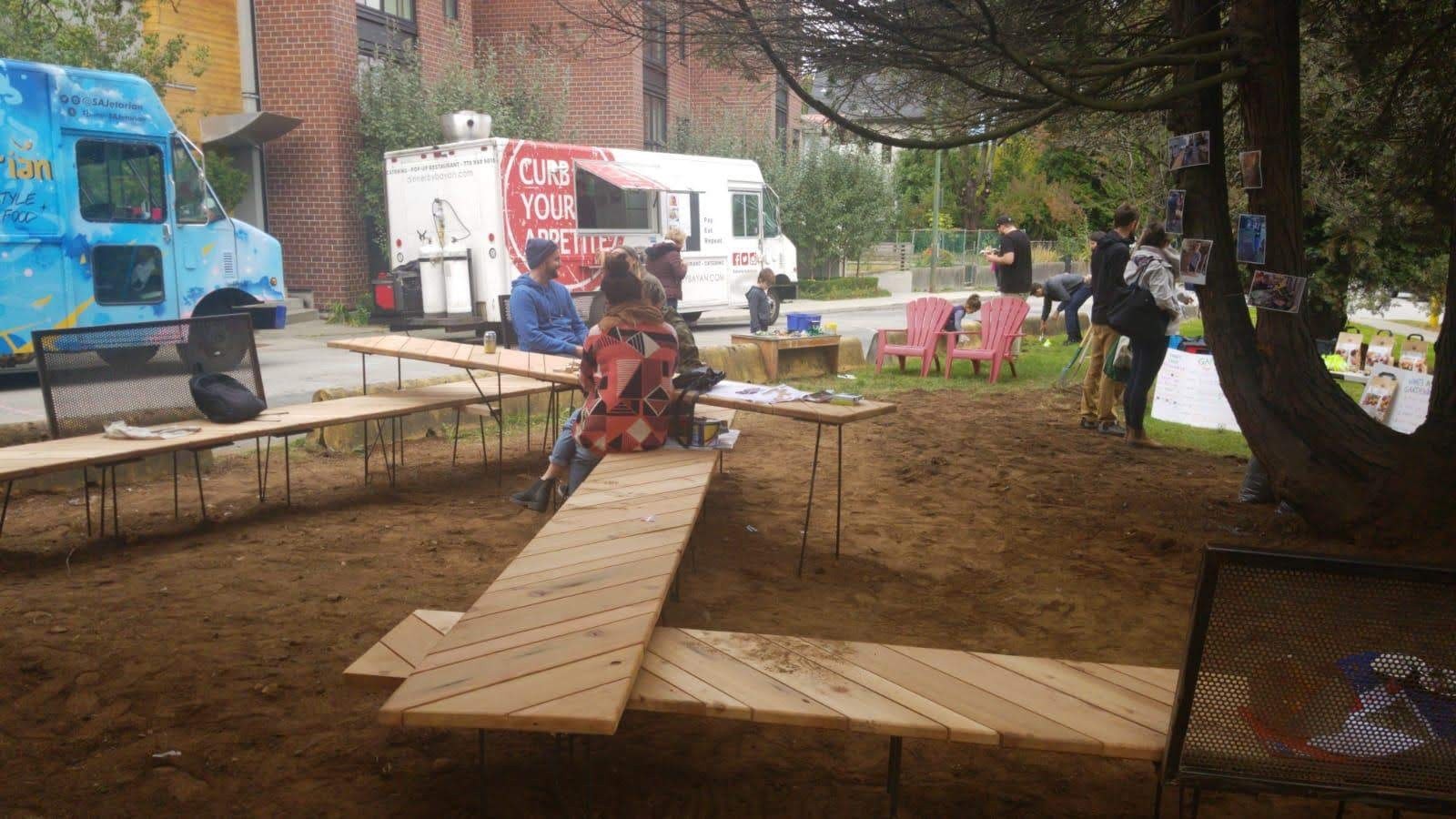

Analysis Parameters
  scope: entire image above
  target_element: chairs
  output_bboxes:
[942,294,1030,384]
[875,297,954,379]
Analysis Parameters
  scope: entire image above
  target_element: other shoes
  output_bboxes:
[1098,420,1125,436]
[1122,435,1163,448]
[1081,419,1099,428]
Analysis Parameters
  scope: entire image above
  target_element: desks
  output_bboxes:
[343,607,1455,818]
[326,333,899,579]
[374,402,739,816]
[729,333,839,382]
[0,375,581,557]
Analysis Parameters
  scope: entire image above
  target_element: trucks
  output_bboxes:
[383,135,799,351]
[1,56,288,376]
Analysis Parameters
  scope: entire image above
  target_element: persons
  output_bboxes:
[986,217,1032,357]
[1080,203,1141,438]
[943,293,981,350]
[645,226,688,311]
[509,238,592,359]
[588,246,702,373]
[745,267,774,334]
[1083,230,1106,283]
[1031,273,1092,345]
[509,252,678,514]
[1165,133,1300,311]
[1122,222,1194,448]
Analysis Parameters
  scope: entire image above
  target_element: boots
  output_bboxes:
[508,474,555,512]
[558,483,569,499]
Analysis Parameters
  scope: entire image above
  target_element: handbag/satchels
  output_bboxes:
[1104,257,1161,336]
[1102,334,1133,383]
[667,397,694,437]
[672,365,726,394]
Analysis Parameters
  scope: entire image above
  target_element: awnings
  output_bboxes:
[197,109,304,148]
[576,161,707,193]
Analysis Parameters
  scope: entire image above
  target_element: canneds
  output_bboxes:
[484,331,496,353]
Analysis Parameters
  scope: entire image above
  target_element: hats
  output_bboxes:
[1030,282,1041,295]
[526,238,558,271]
[994,214,1012,226]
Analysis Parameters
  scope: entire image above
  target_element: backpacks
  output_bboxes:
[189,370,269,425]
